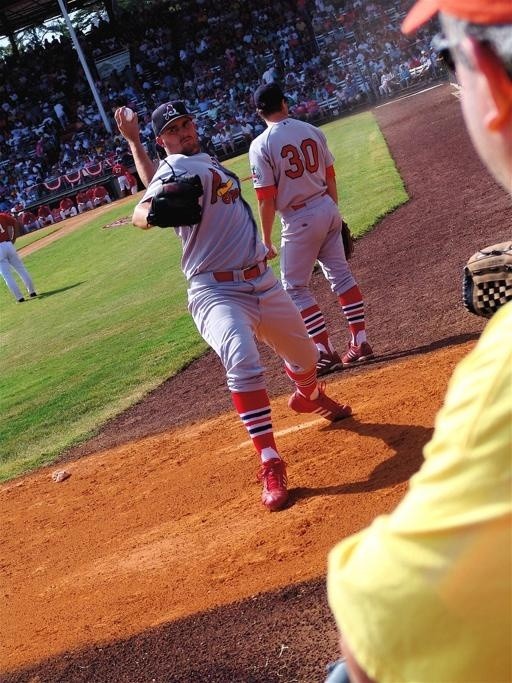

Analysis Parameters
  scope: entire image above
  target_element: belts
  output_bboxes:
[214,257,267,281]
[291,191,328,211]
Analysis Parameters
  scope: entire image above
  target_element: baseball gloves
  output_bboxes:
[341,220,354,260]
[146,175,204,228]
[462,241,512,319]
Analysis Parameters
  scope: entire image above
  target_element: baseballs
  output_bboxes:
[124,107,133,122]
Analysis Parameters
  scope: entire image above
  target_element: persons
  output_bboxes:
[113,101,351,509]
[325,0,512,683]
[248,83,374,377]
[0,213,37,301]
[0,0,451,232]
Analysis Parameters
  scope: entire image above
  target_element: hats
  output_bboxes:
[151,101,196,137]
[401,0,512,36]
[254,84,288,108]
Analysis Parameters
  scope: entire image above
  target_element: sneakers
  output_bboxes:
[257,458,289,511]
[342,340,374,365]
[288,378,352,421]
[316,350,343,377]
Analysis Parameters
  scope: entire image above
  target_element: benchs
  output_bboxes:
[0,2,451,229]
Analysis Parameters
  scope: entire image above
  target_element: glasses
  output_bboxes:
[430,30,490,72]
